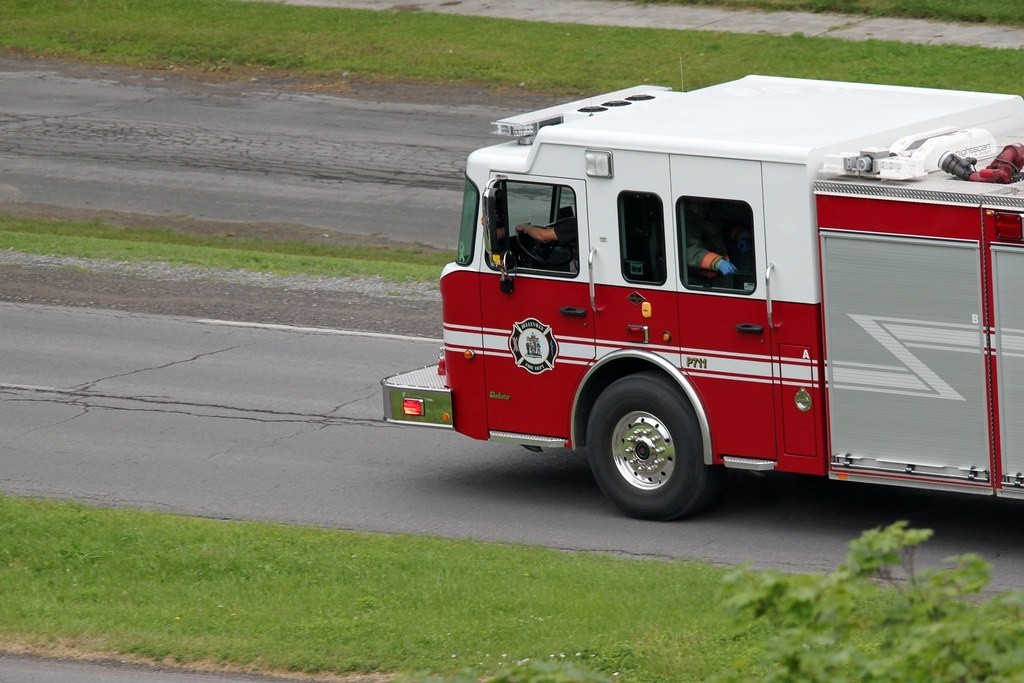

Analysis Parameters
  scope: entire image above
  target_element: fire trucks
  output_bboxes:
[380,75,1024,525]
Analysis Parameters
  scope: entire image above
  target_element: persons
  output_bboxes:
[662,198,746,290]
[515,219,578,272]
